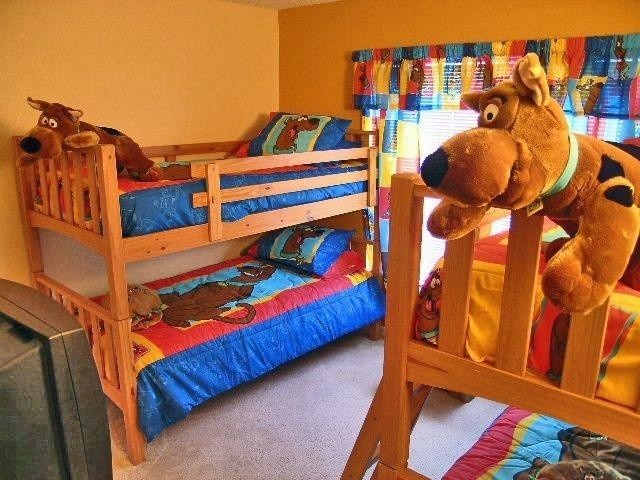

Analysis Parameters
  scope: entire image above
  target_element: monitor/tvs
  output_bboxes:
[0,277,114,480]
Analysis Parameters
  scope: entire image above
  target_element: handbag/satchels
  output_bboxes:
[101,283,163,330]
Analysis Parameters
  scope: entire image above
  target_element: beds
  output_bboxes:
[8,112,378,466]
[341,169,640,479]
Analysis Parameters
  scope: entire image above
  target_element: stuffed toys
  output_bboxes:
[420,53,640,316]
[16,96,162,182]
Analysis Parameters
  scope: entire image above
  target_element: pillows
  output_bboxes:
[247,223,363,279]
[231,113,359,167]
